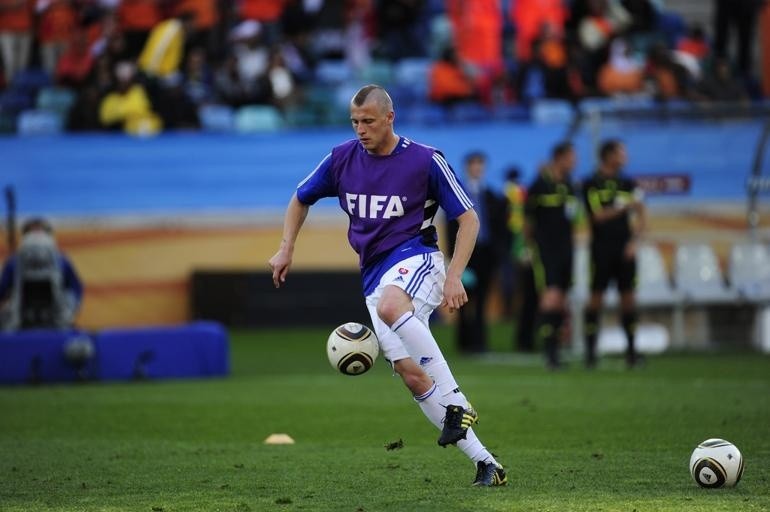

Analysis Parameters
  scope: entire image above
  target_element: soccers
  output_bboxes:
[327,322,379,375]
[690,438,744,488]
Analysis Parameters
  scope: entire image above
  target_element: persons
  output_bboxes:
[444,152,501,354]
[522,142,574,368]
[500,161,538,353]
[1,1,770,137]
[270,84,507,489]
[581,138,648,369]
[0,216,83,332]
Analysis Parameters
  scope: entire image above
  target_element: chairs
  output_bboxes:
[567,240,769,355]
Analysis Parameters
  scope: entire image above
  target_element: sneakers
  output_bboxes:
[437,399,480,447]
[470,455,508,486]
